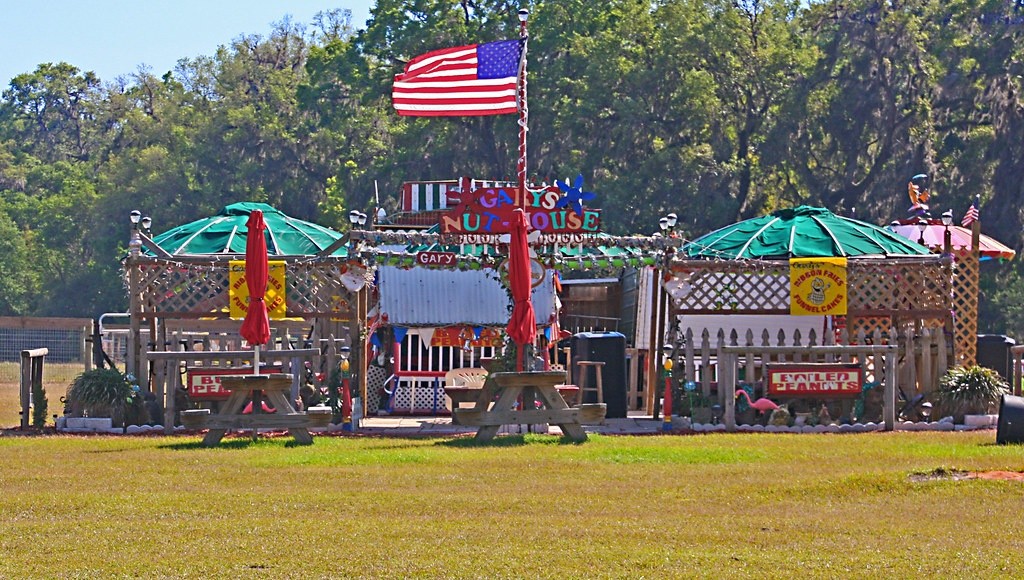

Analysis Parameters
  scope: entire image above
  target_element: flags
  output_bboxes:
[392,35,528,117]
[961,196,979,228]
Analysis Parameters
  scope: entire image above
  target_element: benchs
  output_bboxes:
[454,407,494,439]
[181,409,220,444]
[568,403,608,442]
[296,407,333,444]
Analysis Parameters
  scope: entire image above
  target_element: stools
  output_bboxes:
[577,360,606,404]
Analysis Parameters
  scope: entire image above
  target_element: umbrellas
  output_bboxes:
[240,210,271,376]
[506,208,536,409]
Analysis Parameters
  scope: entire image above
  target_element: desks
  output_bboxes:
[474,371,590,444]
[563,347,639,408]
[146,348,322,436]
[200,373,313,446]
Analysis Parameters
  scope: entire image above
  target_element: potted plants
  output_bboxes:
[68,367,132,426]
[939,365,1012,421]
[685,392,719,425]
[735,385,756,425]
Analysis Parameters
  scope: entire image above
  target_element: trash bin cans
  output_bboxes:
[572,332,628,420]
[976,334,1015,382]
[996,394,1024,444]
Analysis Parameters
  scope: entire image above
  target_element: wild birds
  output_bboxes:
[735,390,779,426]
